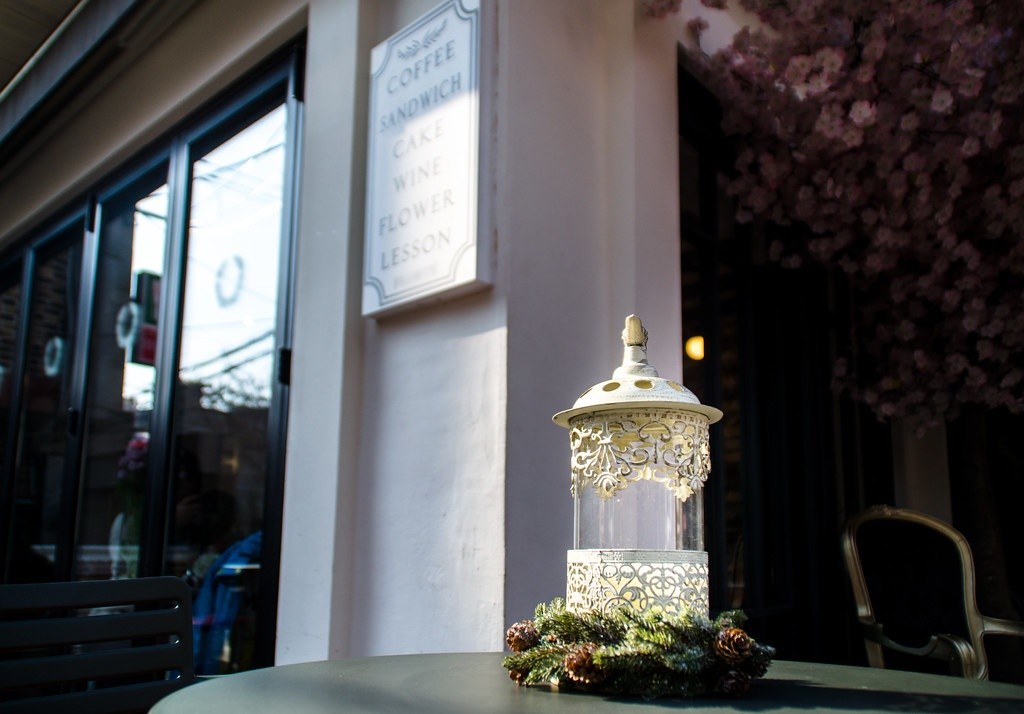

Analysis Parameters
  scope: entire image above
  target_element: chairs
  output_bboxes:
[839,505,1024,682]
[0,574,194,714]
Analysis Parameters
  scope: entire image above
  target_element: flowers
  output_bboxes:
[635,0,1024,442]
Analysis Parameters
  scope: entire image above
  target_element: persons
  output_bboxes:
[1,365,87,702]
[163,432,239,555]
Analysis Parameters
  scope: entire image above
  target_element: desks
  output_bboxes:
[148,650,1024,714]
[224,563,261,571]
